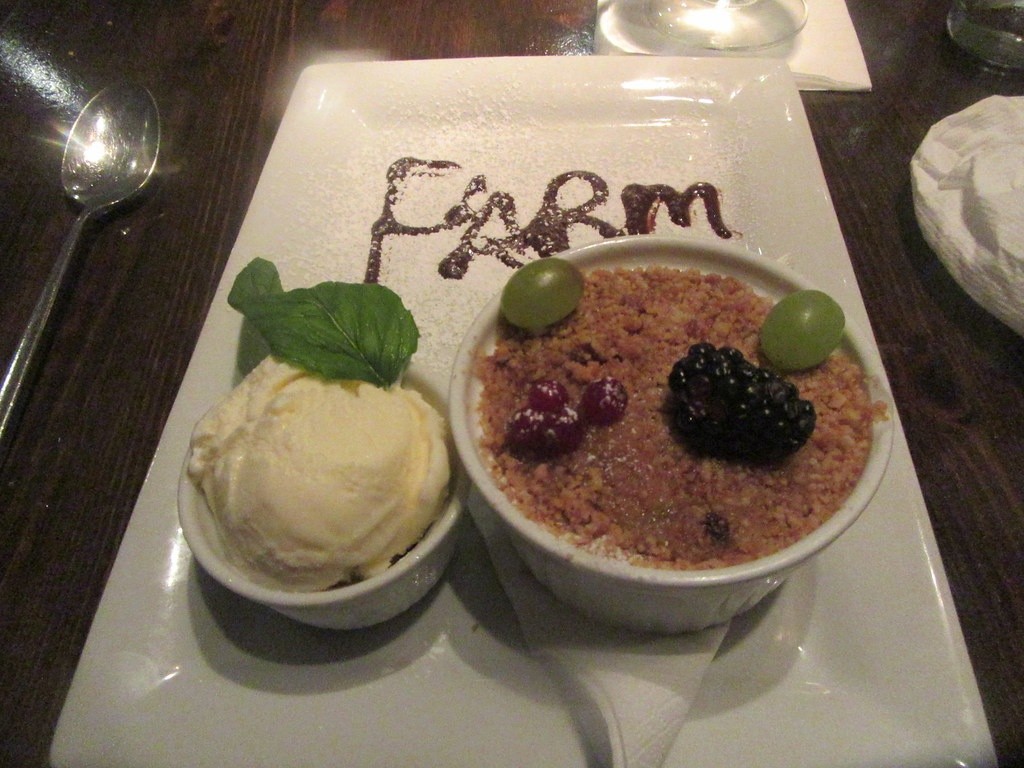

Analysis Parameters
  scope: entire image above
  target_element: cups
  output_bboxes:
[940,0,1024,82]
[175,361,466,630]
[449,235,894,634]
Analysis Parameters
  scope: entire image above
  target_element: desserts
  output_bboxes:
[177,345,472,631]
[447,234,895,634]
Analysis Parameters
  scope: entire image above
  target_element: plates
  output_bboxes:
[48,56,1000,768]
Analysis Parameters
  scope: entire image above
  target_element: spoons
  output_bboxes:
[0,79,163,468]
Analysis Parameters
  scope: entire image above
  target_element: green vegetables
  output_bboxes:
[228,256,421,390]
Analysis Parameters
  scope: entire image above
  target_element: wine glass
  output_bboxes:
[643,0,809,51]
